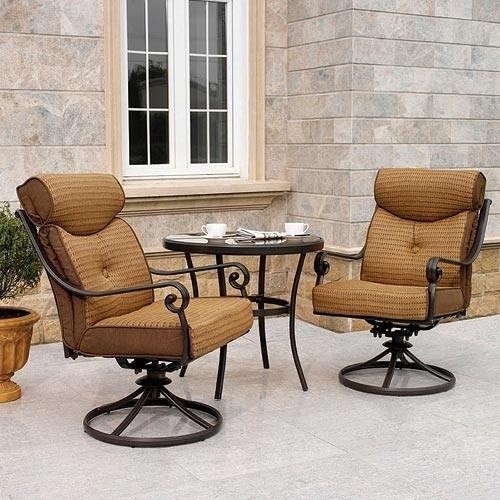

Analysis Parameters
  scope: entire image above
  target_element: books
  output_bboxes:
[236,238,286,246]
[235,225,282,241]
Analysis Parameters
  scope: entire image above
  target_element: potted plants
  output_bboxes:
[0,201,39,403]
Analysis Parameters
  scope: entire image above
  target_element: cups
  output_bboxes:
[285,223,309,236]
[201,224,228,237]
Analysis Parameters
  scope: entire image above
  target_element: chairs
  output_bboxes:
[311,167,492,398]
[14,172,254,448]
